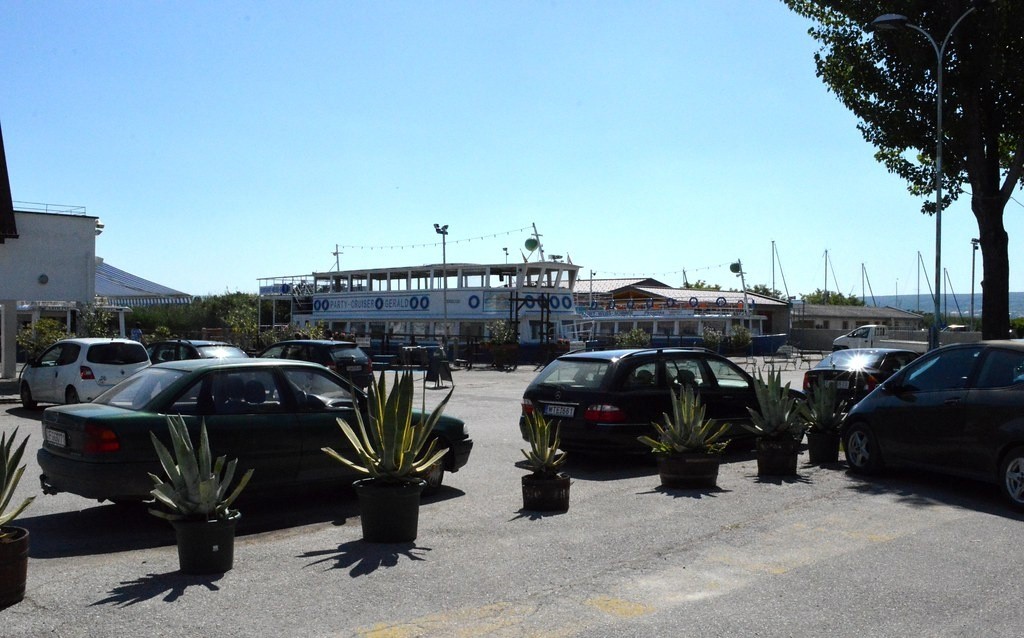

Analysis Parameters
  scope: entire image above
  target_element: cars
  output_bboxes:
[19,337,153,411]
[144,337,250,367]
[940,324,971,333]
[253,339,375,392]
[839,339,1024,511]
[37,357,473,532]
[803,347,922,432]
[519,344,812,466]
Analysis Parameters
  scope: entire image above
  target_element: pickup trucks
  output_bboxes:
[832,324,930,358]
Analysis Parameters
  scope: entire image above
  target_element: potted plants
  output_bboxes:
[796,374,848,463]
[637,381,732,488]
[1,426,37,608]
[520,408,570,514]
[144,411,256,573]
[322,365,456,543]
[741,364,813,478]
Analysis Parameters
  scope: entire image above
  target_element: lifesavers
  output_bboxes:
[716,296,727,307]
[608,299,616,309]
[314,299,329,310]
[627,298,634,309]
[591,301,598,310]
[375,298,384,310]
[666,297,675,307]
[646,298,654,308]
[468,295,480,308]
[689,297,698,307]
[524,294,573,309]
[410,296,429,309]
[281,283,290,294]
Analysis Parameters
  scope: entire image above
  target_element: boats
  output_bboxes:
[570,296,789,356]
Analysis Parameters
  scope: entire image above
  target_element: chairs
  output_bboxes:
[637,369,654,386]
[216,374,280,413]
[402,341,568,372]
[676,369,699,389]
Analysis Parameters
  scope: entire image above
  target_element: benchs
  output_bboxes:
[718,355,757,375]
[799,351,824,369]
[761,353,797,372]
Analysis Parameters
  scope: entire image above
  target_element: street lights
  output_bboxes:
[433,223,449,351]
